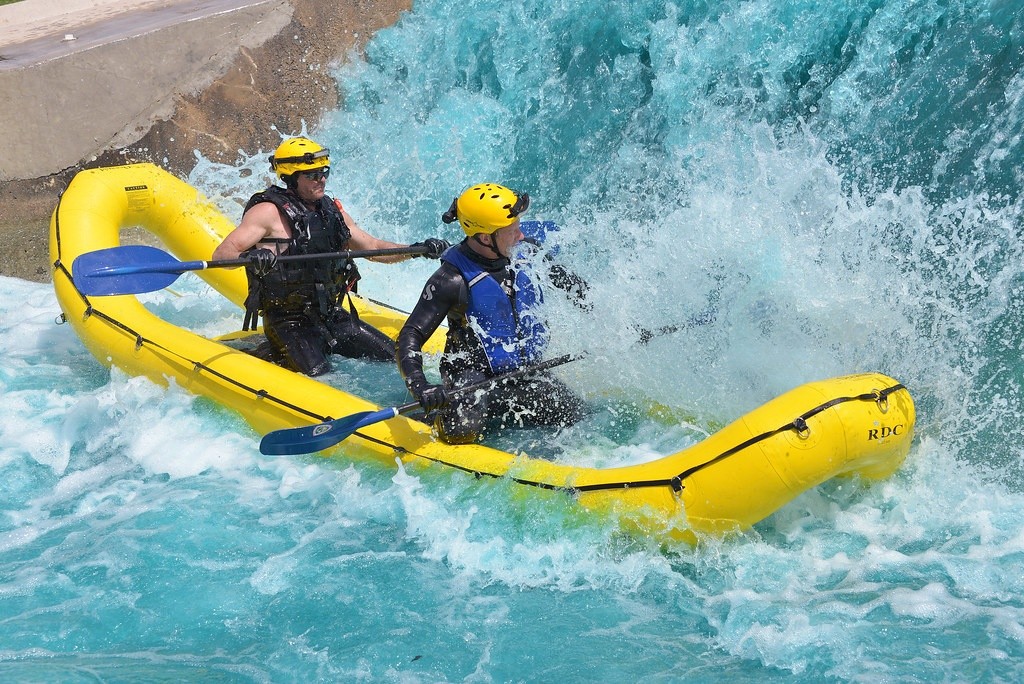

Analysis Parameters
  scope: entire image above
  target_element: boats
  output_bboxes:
[45,161,916,548]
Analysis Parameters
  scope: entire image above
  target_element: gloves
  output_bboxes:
[410,238,451,260]
[409,379,450,414]
[628,321,653,346]
[237,248,277,276]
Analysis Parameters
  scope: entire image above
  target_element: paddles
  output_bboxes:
[256,290,781,460]
[68,217,564,299]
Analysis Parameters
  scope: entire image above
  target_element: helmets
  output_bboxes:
[272,138,330,180]
[457,183,529,237]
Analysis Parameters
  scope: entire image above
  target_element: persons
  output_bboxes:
[210,138,451,378]
[396,183,651,446]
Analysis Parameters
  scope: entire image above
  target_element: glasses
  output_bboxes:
[301,165,330,180]
